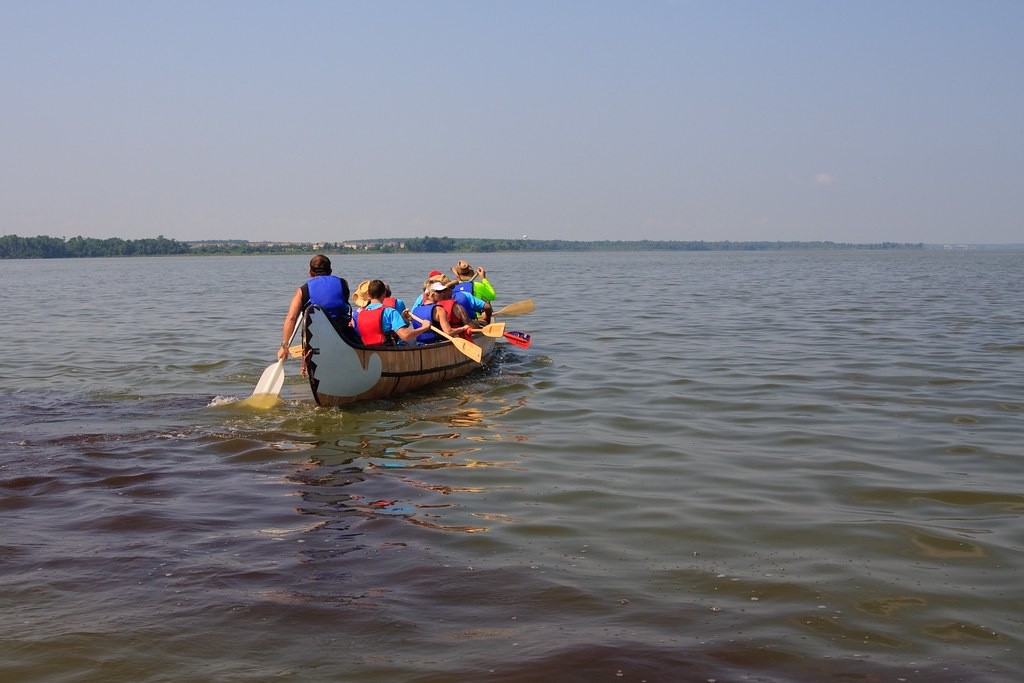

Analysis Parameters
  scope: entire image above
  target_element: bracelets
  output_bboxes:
[281,341,289,349]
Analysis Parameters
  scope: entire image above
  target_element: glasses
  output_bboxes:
[449,285,455,289]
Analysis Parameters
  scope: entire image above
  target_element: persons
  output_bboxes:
[411,261,496,346]
[277,254,350,361]
[348,280,431,346]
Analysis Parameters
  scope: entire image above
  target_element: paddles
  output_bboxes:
[478,322,531,348]
[403,309,483,363]
[491,299,535,316]
[451,322,506,338]
[248,310,304,408]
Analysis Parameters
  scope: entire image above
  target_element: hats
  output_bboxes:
[424,271,459,295]
[451,260,474,280]
[352,280,371,307]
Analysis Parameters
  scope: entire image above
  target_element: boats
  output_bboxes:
[299,300,498,409]
